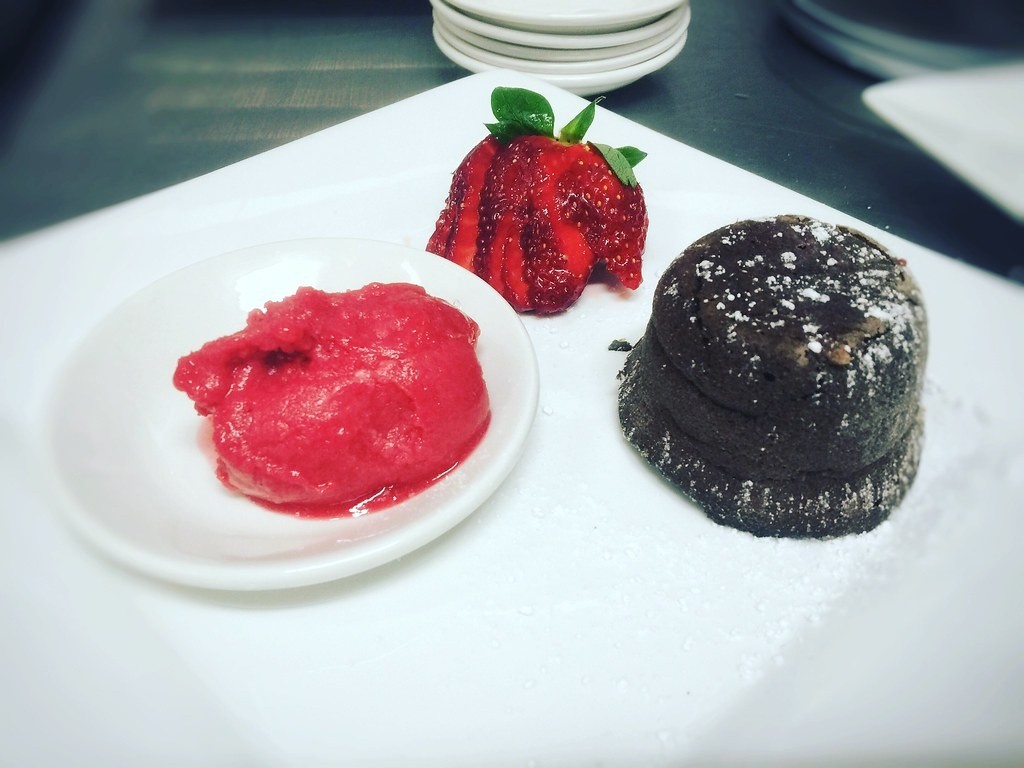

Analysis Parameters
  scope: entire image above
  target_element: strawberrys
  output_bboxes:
[422,85,648,318]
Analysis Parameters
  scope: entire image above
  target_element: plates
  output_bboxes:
[778,0,1024,80]
[431,0,692,88]
[861,64,1023,223]
[0,64,1023,768]
[53,238,542,588]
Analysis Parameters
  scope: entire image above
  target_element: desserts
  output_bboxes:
[171,278,491,518]
[616,214,928,540]
